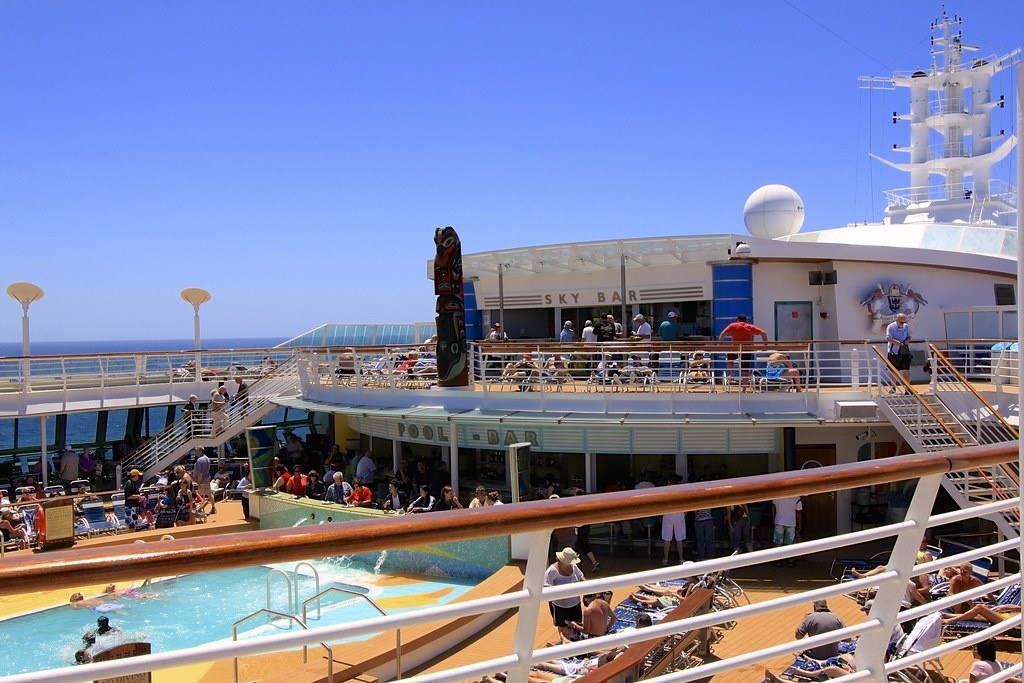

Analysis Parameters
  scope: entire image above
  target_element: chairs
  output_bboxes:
[0,432,362,556]
[173,351,800,392]
[468,522,1022,683]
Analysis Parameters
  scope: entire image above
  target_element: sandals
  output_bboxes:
[788,561,794,567]
[775,561,780,566]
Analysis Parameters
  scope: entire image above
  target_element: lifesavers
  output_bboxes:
[34,505,45,545]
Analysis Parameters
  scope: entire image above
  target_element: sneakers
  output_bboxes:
[209,508,217,514]
[888,388,896,394]
[904,388,918,395]
[679,560,687,565]
[661,559,669,566]
[592,562,600,577]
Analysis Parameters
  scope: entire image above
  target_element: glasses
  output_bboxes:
[294,471,301,472]
[311,475,317,477]
[896,319,906,324]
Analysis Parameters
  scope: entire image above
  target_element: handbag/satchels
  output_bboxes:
[888,342,901,370]
[899,345,913,370]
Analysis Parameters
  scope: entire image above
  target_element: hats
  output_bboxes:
[78,484,86,490]
[668,311,679,318]
[606,353,612,357]
[555,547,582,564]
[631,354,641,359]
[564,321,573,329]
[130,469,144,477]
[633,314,644,321]
[494,322,501,327]
[693,351,705,358]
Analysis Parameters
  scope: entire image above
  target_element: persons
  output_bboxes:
[1,433,1023,683]
[559,319,581,368]
[630,313,654,347]
[612,354,652,384]
[657,311,685,342]
[685,349,712,384]
[524,349,572,394]
[717,315,769,394]
[588,352,619,383]
[498,353,539,380]
[607,315,625,339]
[484,322,509,376]
[765,347,805,394]
[577,319,599,364]
[884,312,920,396]
[592,314,617,345]
[171,320,438,434]
